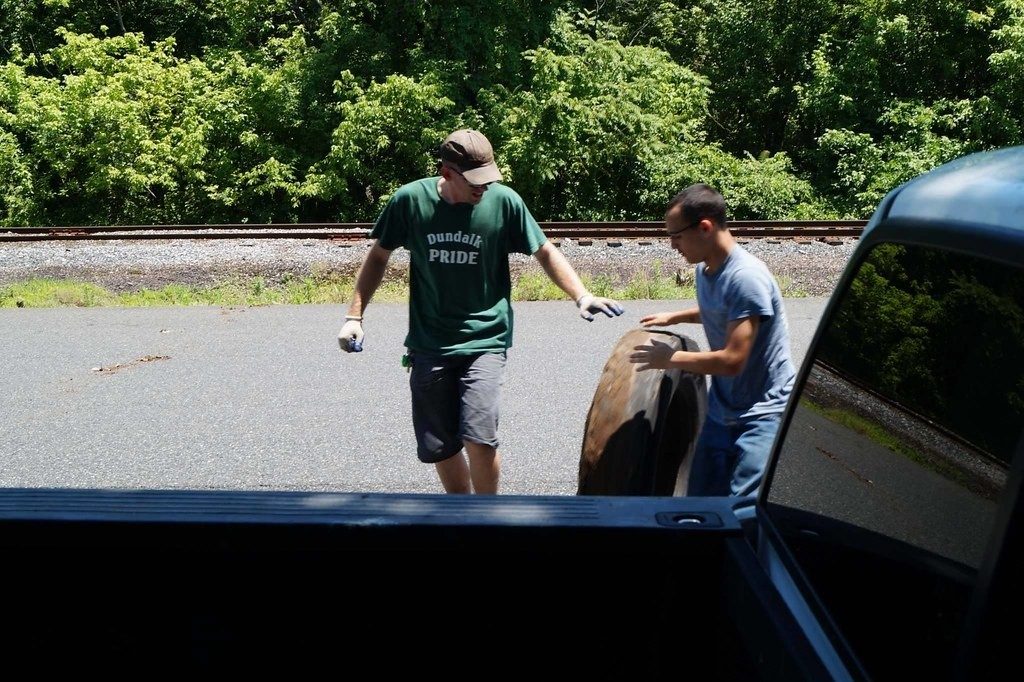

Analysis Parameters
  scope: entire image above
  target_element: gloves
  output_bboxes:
[337,316,365,353]
[576,293,625,322]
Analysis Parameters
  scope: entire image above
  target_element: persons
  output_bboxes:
[337,129,624,494]
[628,183,796,498]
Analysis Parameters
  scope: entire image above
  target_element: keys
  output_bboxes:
[405,349,413,373]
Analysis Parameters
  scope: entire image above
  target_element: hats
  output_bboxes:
[440,129,503,185]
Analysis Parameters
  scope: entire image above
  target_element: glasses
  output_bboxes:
[667,221,700,240]
[446,165,494,189]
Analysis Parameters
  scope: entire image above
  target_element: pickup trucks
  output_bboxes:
[0,148,1024,682]
